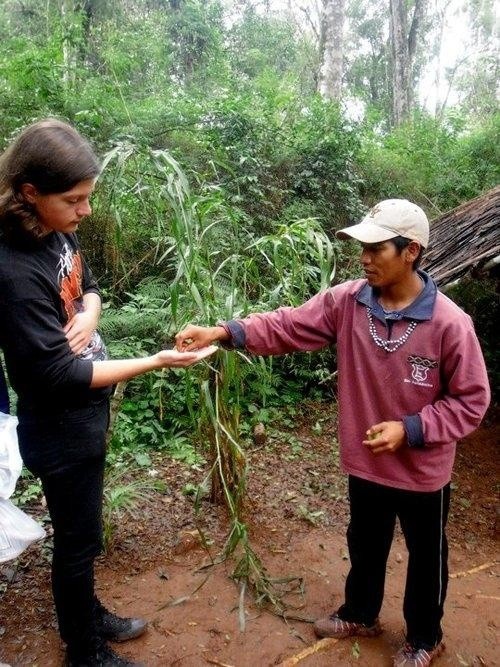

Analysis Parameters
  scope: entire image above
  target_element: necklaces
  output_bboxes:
[365,306,419,353]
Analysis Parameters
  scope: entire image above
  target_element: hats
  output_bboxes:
[335,198,429,249]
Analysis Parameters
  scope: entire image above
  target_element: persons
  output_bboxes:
[0,117,219,667]
[175,196,491,667]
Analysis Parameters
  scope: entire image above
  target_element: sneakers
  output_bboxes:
[393,637,447,667]
[92,599,147,641]
[314,605,382,638]
[67,636,144,667]
